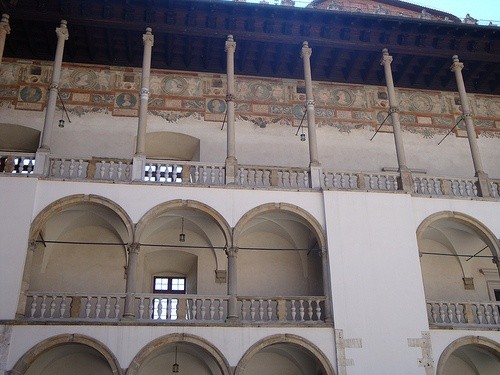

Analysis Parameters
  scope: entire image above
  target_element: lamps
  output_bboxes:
[179,217,186,243]
[58,107,65,128]
[172,346,179,373]
[300,123,306,142]
